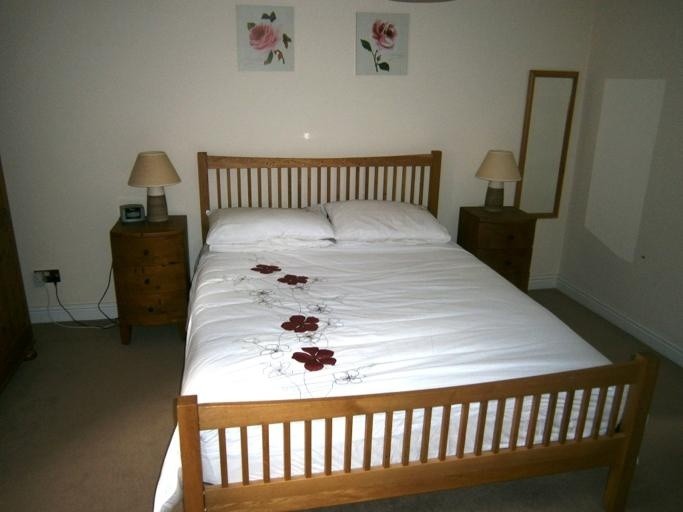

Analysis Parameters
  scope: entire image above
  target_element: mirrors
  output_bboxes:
[513,69,580,219]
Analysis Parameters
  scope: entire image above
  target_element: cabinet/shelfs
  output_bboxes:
[0,159,39,393]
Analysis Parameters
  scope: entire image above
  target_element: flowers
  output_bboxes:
[359,20,397,72]
[246,10,291,64]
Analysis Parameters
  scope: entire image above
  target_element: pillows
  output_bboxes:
[206,205,335,245]
[324,200,452,242]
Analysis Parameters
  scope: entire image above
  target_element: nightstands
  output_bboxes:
[457,206,536,295]
[110,214,190,346]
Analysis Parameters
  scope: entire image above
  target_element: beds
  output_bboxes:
[176,149,660,512]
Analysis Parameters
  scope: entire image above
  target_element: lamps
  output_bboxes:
[127,150,182,222]
[475,150,521,212]
